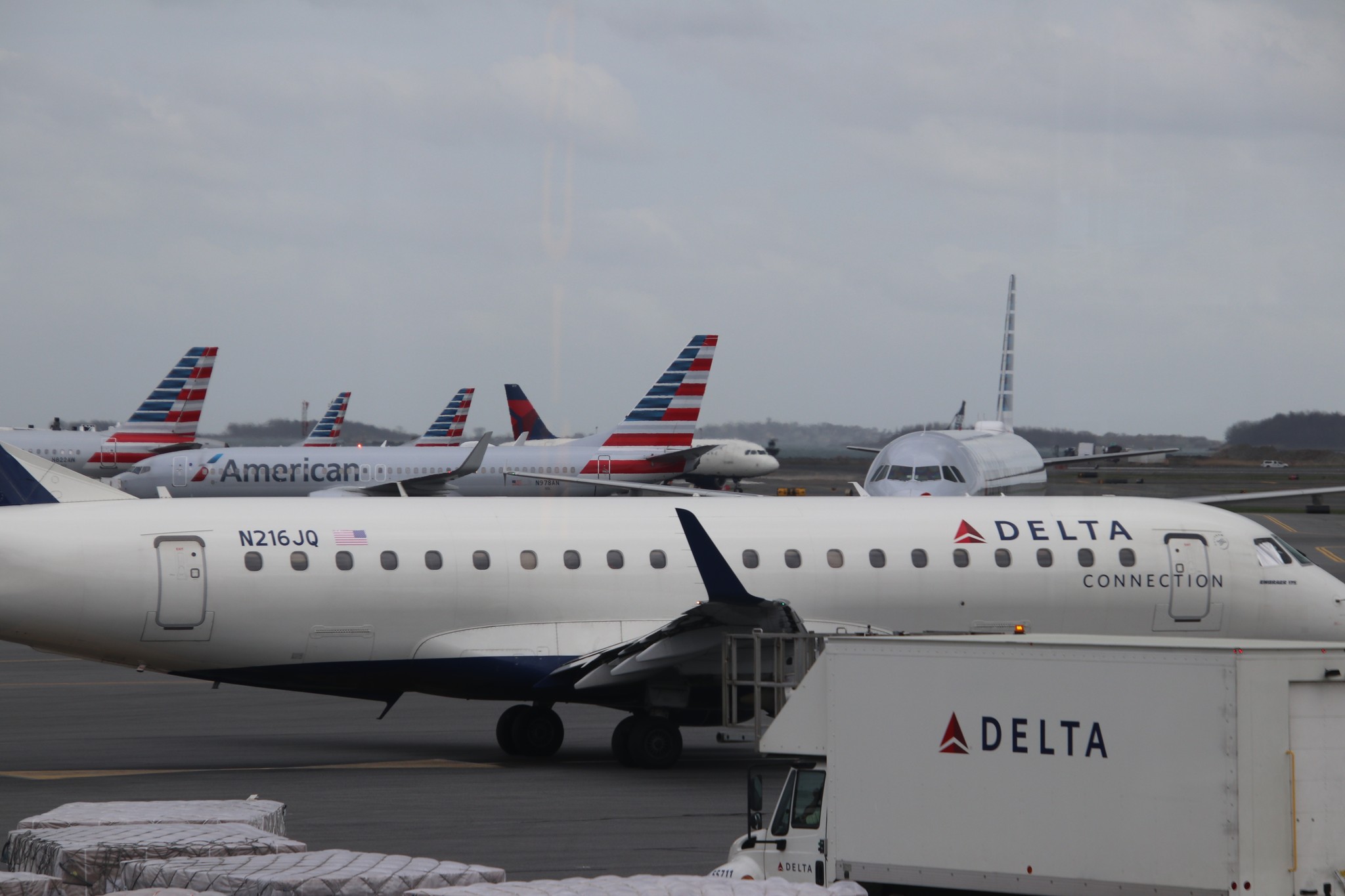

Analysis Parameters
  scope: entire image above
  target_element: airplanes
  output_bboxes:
[844,273,1181,497]
[0,334,782,496]
[0,436,1345,775]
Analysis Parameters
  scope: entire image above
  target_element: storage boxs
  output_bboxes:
[0,797,873,896]
